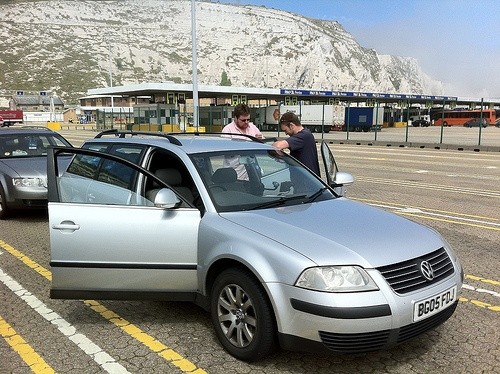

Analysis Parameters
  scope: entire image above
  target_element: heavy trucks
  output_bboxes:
[249,106,385,133]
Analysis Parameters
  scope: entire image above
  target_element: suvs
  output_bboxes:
[46,129,464,362]
[0,125,82,218]
[463,117,487,128]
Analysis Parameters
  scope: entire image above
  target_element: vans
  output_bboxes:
[409,115,430,124]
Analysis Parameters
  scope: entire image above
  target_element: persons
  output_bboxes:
[221,104,266,182]
[272,113,322,193]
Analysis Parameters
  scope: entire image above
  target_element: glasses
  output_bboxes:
[237,118,251,122]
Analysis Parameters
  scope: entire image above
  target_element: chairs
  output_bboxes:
[147,169,194,206]
[210,168,249,192]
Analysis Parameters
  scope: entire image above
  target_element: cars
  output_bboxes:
[412,120,429,127]
[495,120,500,127]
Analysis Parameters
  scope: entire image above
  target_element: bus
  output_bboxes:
[432,109,496,127]
